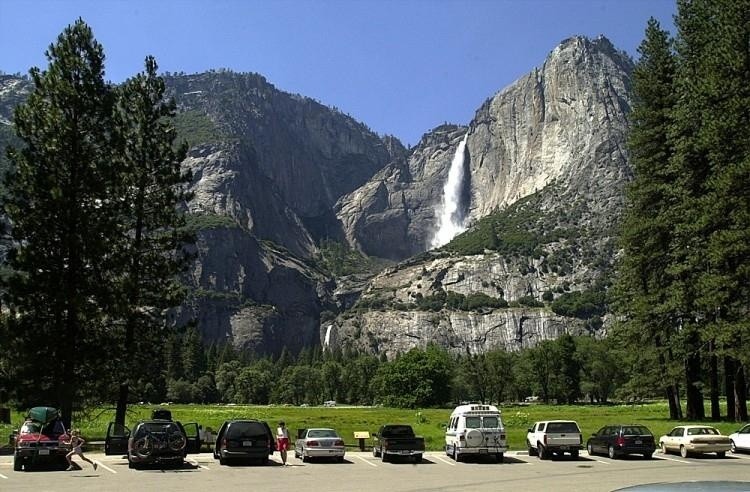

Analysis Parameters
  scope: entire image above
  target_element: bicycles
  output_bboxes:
[135,424,186,459]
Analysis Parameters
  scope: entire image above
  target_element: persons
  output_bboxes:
[198,425,204,446]
[206,426,213,448]
[62,429,98,471]
[275,421,291,466]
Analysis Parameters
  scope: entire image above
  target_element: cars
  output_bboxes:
[659,424,731,459]
[294,427,345,462]
[586,423,657,459]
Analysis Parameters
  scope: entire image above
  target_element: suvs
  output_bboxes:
[104,407,202,467]
[13,406,73,470]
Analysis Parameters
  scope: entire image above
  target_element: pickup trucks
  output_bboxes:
[372,424,426,463]
[523,419,583,459]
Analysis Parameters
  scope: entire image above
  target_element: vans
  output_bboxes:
[212,417,274,464]
[726,423,750,453]
[445,403,507,462]
